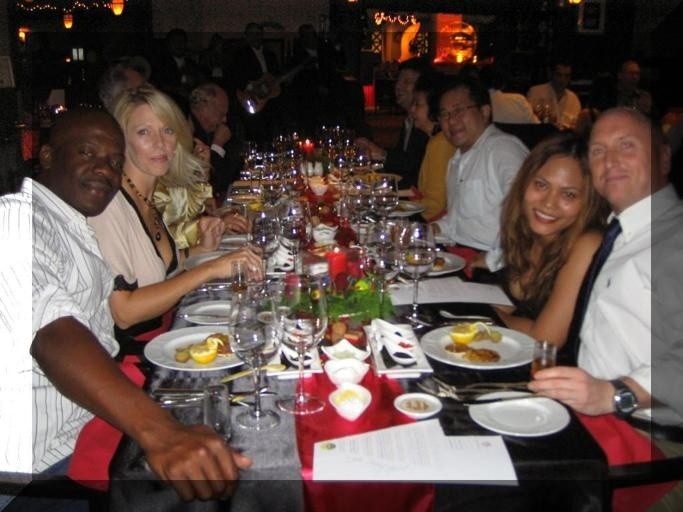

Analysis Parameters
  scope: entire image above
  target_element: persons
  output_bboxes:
[488,140,602,355]
[353,56,638,281]
[99,22,326,246]
[527,107,681,463]
[93,86,265,357]
[1,105,252,511]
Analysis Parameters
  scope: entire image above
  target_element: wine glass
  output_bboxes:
[227,125,433,430]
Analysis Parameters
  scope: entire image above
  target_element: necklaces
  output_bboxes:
[119,170,168,241]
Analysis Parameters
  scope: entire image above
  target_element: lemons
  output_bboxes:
[450,325,477,344]
[189,345,218,363]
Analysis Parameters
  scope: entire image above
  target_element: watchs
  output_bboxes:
[609,380,637,420]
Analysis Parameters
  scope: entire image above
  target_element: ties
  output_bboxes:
[556,218,622,366]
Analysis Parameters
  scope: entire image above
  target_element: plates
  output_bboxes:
[467,390,571,438]
[395,246,465,277]
[144,232,262,371]
[419,323,540,368]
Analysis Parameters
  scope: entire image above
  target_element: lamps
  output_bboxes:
[64,12,73,30]
[112,0,125,17]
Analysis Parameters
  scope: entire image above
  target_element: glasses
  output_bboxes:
[436,102,480,121]
